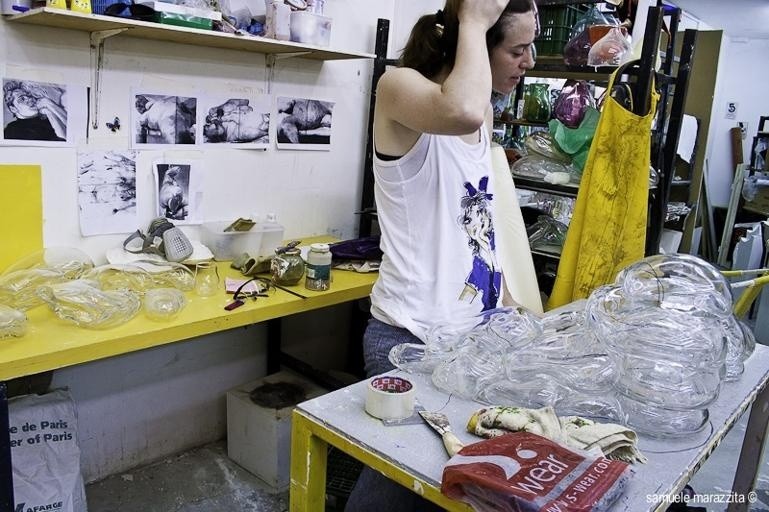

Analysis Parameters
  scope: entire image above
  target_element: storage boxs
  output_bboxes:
[224,366,331,491]
[290,10,335,47]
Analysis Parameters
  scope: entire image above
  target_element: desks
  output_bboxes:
[288,294,769,511]
[0,234,381,511]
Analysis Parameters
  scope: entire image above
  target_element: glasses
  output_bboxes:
[102,3,156,20]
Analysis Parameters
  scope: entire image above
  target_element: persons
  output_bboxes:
[21,82,69,127]
[343,1,535,512]
[3,83,66,141]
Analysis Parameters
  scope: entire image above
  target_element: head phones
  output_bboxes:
[610,59,662,114]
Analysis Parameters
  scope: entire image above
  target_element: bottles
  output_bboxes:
[305,243,335,292]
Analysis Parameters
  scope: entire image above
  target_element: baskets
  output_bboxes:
[92,0,132,16]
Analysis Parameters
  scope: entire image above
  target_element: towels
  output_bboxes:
[464,395,649,471]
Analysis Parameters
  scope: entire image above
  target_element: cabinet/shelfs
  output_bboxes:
[718,115,769,271]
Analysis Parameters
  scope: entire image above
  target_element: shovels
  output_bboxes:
[274,239,302,255]
[418,409,465,458]
